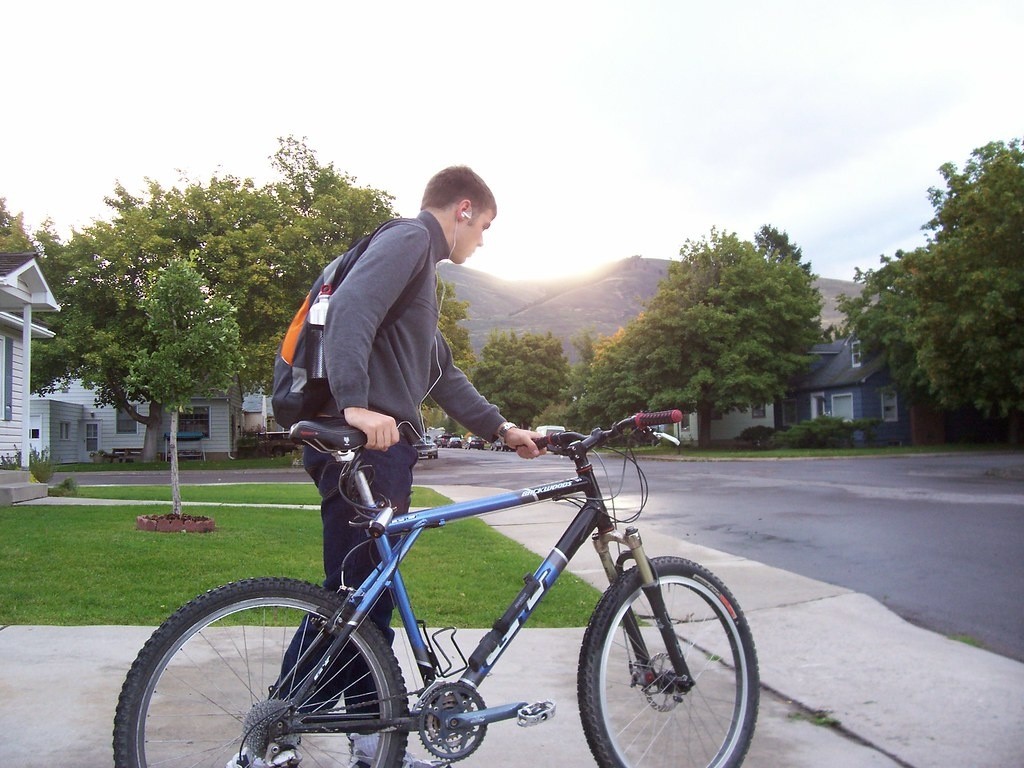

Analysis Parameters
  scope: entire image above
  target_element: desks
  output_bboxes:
[110,447,145,463]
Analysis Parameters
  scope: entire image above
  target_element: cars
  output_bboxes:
[491,438,518,451]
[412,435,438,460]
[434,433,452,448]
[111,409,762,768]
[446,436,463,448]
[465,436,486,450]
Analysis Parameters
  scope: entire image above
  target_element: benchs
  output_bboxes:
[120,454,137,463]
[167,448,203,462]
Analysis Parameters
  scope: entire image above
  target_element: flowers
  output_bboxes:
[89,449,108,457]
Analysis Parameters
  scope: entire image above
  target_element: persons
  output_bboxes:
[227,166,547,767]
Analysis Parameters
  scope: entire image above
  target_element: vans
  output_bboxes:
[535,425,566,438]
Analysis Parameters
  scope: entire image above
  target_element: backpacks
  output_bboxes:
[270,219,431,431]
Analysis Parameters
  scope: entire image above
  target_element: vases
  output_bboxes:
[93,456,105,464]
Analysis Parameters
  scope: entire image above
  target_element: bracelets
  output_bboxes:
[499,423,516,442]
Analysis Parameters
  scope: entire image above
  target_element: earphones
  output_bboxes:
[461,212,471,219]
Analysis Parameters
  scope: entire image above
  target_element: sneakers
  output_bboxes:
[348,752,453,768]
[226,747,252,768]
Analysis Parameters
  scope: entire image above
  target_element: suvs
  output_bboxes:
[238,430,297,460]
